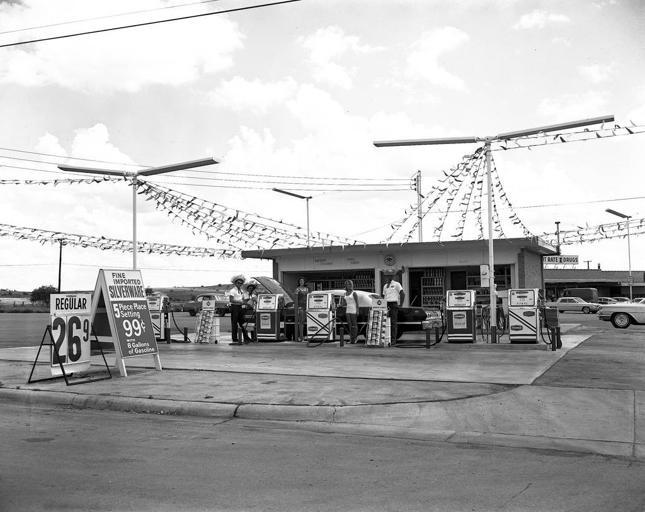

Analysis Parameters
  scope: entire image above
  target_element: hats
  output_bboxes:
[245,281,257,290]
[383,269,395,276]
[232,274,244,283]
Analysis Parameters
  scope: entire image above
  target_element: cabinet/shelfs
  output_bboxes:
[466,266,510,316]
[307,278,375,292]
[421,275,444,308]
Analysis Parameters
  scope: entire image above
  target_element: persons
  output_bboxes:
[342,280,360,344]
[382,276,405,345]
[227,275,252,342]
[243,284,258,342]
[294,278,309,341]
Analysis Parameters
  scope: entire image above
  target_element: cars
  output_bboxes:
[598,298,645,329]
[632,297,644,303]
[240,274,443,346]
[545,297,602,314]
[182,293,232,317]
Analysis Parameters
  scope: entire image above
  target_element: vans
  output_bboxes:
[613,295,629,302]
[599,296,617,305]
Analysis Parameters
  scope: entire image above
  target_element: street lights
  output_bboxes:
[584,260,594,270]
[553,220,561,251]
[371,113,616,344]
[56,156,221,270]
[272,187,311,246]
[56,236,66,294]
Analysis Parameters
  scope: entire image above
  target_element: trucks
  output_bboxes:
[562,287,599,304]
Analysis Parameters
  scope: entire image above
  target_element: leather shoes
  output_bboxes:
[390,340,395,345]
[244,339,254,344]
[346,341,354,344]
[354,337,358,343]
[232,339,237,342]
